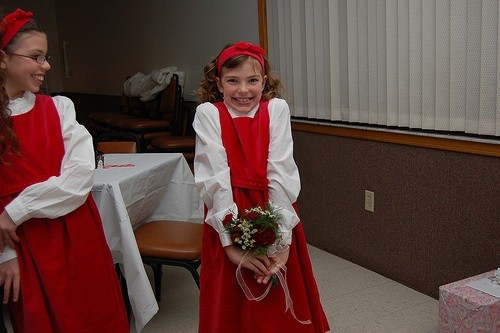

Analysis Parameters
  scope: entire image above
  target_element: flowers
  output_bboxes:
[219,199,284,290]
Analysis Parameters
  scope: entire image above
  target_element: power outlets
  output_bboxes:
[364,190,375,212]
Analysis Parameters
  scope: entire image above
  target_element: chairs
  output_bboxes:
[135,220,202,302]
[88,74,195,160]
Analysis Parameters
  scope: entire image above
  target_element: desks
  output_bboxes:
[91,152,183,326]
[438,269,500,333]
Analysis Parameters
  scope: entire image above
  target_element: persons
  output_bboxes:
[192,41,331,333]
[0,9,130,333]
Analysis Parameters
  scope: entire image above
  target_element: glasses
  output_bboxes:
[6,52,52,64]
